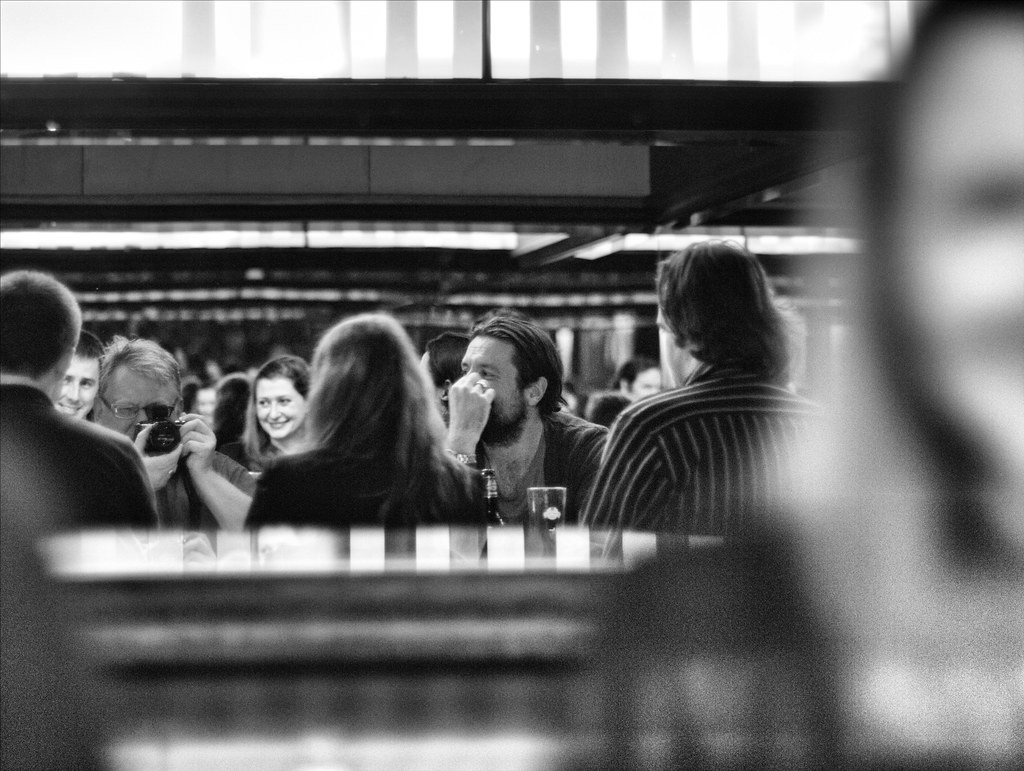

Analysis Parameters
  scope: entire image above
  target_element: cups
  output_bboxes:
[527,486,567,532]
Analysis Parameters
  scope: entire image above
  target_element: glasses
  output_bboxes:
[98,394,179,419]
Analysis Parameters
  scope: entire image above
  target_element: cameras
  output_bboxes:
[134,421,184,458]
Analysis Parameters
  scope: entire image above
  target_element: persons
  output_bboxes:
[217,355,315,478]
[418,306,610,528]
[1,268,162,533]
[91,335,259,530]
[245,308,493,527]
[582,2,1024,771]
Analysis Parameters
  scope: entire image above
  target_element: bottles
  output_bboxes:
[480,468,505,527]
